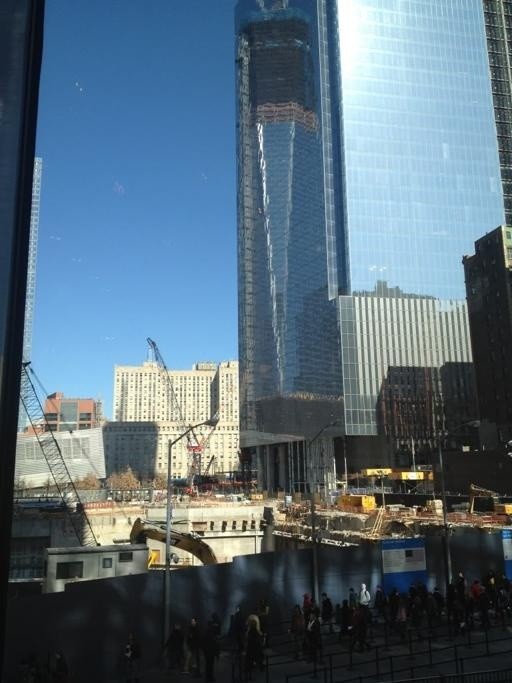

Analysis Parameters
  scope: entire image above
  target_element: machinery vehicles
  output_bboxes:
[130,517,217,566]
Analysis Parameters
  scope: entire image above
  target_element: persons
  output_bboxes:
[164,569,511,683]
[125,631,138,671]
[52,647,68,681]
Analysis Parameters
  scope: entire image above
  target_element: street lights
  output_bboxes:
[437,420,481,584]
[162,415,219,675]
[308,417,341,617]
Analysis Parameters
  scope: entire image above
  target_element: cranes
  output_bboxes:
[335,478,378,514]
[145,337,260,496]
[469,483,512,525]
